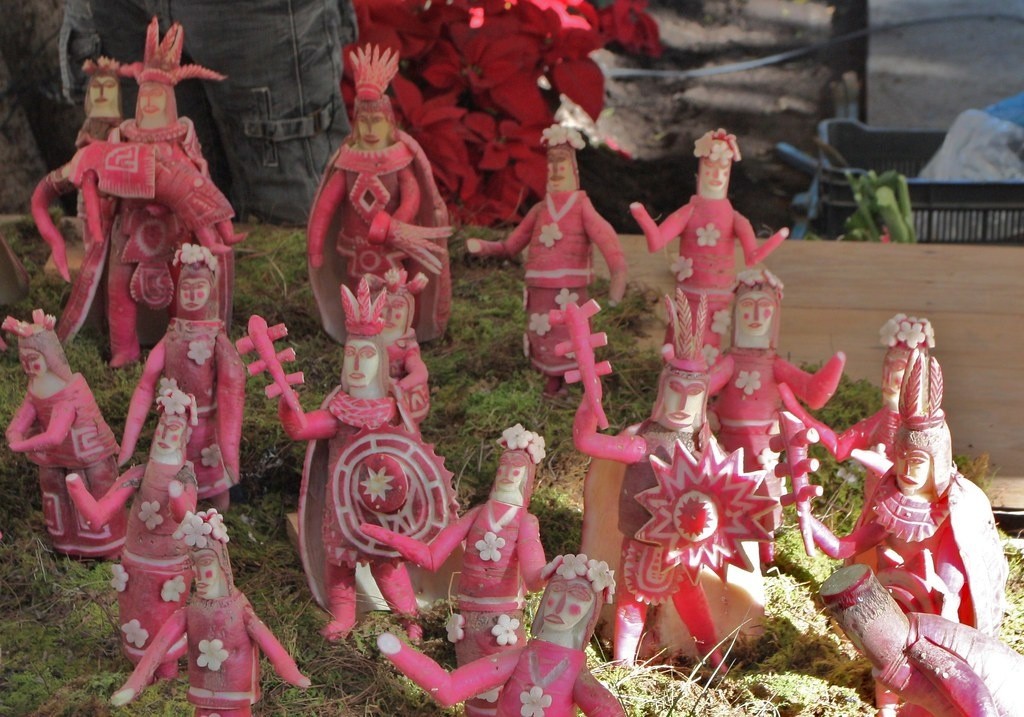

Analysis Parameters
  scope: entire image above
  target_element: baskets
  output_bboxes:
[818,116,1024,245]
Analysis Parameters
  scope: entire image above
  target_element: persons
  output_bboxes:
[1,310,130,561]
[816,563,1024,717]
[466,125,628,398]
[308,41,454,343]
[777,313,935,505]
[119,242,246,514]
[630,126,790,372]
[1,0,357,228]
[703,269,844,563]
[572,362,728,677]
[376,554,625,717]
[108,509,311,717]
[63,379,200,680]
[25,15,247,369]
[277,336,426,646]
[798,420,1007,636]
[360,269,431,425]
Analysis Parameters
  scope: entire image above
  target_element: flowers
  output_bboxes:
[343,0,670,223]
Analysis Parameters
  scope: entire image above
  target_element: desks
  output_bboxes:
[591,233,1023,534]
